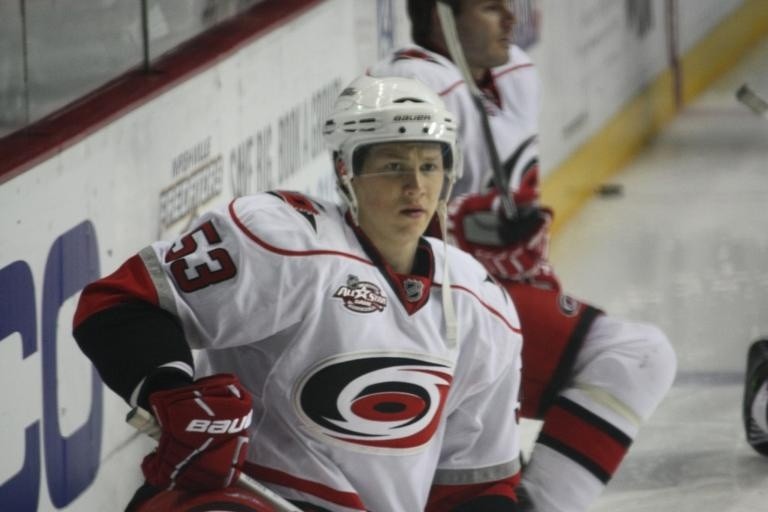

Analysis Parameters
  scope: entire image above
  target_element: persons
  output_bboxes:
[74,65,525,509]
[370,0,681,512]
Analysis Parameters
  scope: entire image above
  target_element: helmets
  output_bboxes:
[323,75,458,226]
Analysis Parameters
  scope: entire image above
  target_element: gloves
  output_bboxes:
[138,373,256,495]
[449,188,555,285]
